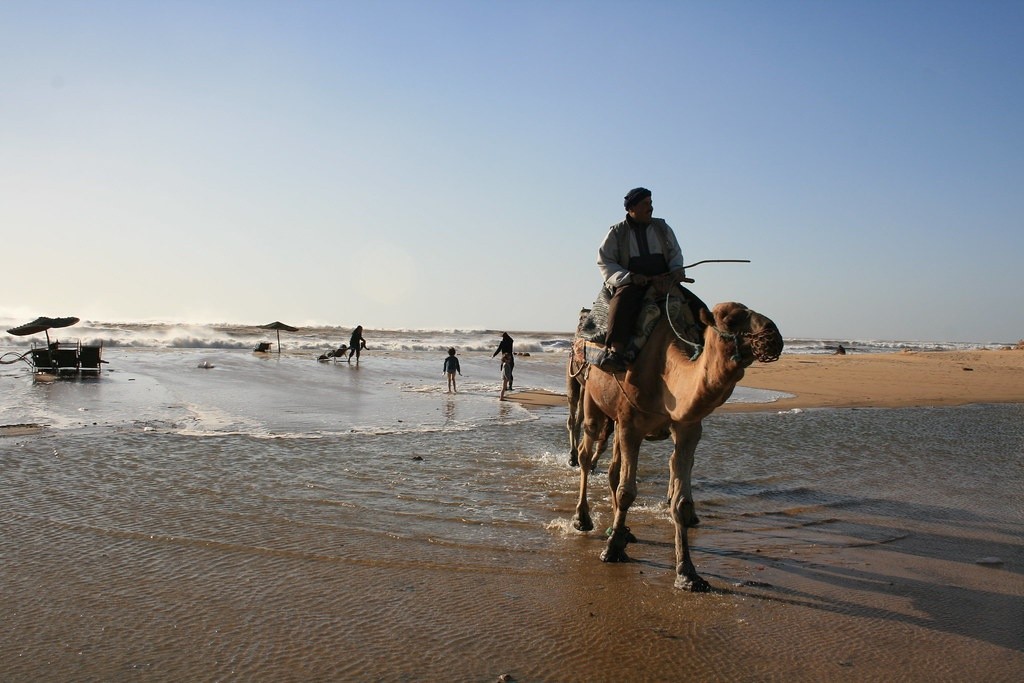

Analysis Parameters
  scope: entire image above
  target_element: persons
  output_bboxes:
[500,352,512,401]
[491,332,514,390]
[834,345,846,355]
[443,347,461,392]
[596,187,685,371]
[348,326,366,366]
[327,344,347,357]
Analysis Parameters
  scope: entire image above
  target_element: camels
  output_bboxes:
[564,299,784,593]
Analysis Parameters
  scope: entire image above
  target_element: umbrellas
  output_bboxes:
[256,321,300,352]
[6,317,80,348]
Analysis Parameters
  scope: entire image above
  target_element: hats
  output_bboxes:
[623,187,651,211]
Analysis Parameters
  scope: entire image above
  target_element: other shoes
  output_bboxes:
[600,351,627,374]
